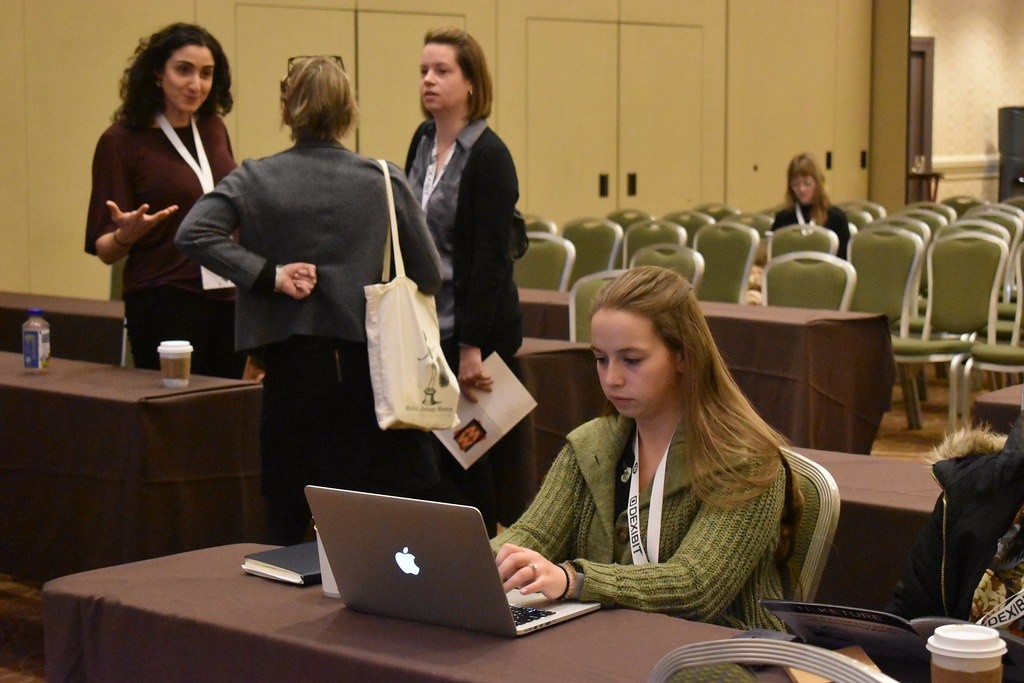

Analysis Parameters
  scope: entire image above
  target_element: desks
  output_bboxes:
[907,169,941,202]
[0,282,1022,683]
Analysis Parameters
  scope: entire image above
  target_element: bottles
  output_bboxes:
[22,308,51,375]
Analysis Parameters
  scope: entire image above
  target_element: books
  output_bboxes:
[759,598,1024,683]
[782,644,882,683]
[242,539,322,587]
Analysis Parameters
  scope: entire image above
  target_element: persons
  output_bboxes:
[172,55,444,546]
[770,152,852,263]
[488,261,803,638]
[83,21,238,376]
[401,24,532,534]
[882,391,1024,642]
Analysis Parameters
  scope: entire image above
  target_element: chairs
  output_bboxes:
[512,191,1024,432]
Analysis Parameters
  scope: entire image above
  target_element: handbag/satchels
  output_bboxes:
[513,210,529,258]
[363,159,461,431]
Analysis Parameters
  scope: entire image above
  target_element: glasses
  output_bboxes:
[287,56,346,80]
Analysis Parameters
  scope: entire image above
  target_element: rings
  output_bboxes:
[528,562,537,578]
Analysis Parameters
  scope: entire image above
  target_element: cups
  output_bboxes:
[314,525,342,598]
[158,341,194,388]
[926,624,1008,683]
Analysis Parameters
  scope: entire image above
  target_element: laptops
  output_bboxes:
[304,486,601,638]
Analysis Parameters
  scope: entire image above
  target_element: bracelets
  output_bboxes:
[557,563,575,603]
[113,232,133,247]
